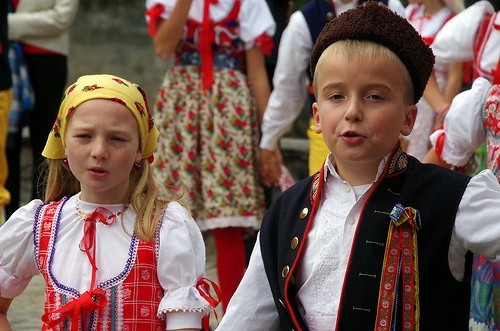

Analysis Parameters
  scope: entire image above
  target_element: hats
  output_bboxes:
[310,3,436,104]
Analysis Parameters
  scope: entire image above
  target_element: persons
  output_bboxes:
[256,0,380,187]
[213,0,500,331]
[145,0,276,269]
[423,47,500,331]
[422,1,500,130]
[0,74,221,331]
[396,0,447,164]
[4,0,78,223]
[0,0,12,228]
